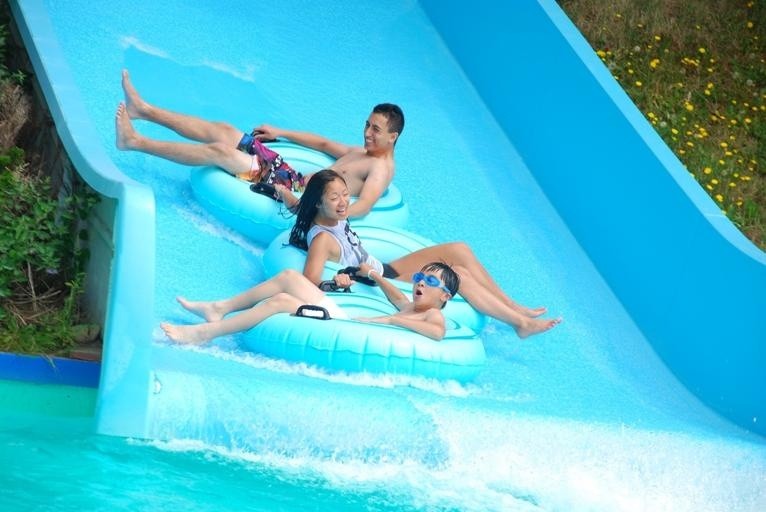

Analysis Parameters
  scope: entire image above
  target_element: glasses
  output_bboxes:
[412,271,452,296]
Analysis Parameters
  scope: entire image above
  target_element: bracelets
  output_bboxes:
[322,284,334,292]
[366,270,380,280]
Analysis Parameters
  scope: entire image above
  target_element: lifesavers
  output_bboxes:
[261,219,491,336]
[188,131,407,244]
[222,292,486,387]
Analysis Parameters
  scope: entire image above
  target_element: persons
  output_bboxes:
[280,170,564,339]
[115,68,406,222]
[157,261,462,346]
